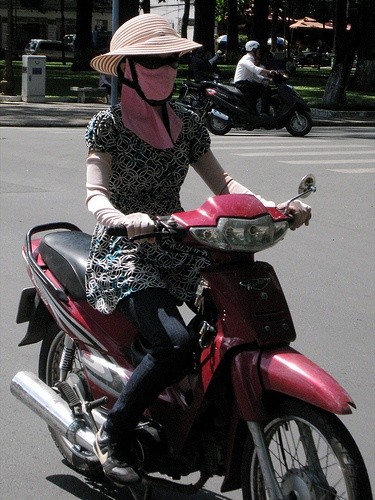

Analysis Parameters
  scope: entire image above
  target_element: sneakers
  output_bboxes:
[92,425,139,485]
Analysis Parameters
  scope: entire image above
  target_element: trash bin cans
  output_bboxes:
[21,54,46,103]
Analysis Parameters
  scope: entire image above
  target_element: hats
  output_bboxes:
[89,13,203,76]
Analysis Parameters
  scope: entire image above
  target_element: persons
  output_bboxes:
[84,14,312,484]
[231,40,278,119]
[187,46,224,83]
[254,55,270,87]
[99,73,111,104]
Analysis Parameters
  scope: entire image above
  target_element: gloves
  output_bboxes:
[121,212,157,245]
[276,198,312,231]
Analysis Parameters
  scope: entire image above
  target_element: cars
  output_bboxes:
[20,33,77,62]
[293,51,331,67]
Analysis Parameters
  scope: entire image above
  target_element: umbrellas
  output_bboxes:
[216,35,242,49]
[224,1,351,54]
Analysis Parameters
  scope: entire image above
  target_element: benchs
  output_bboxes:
[70,86,108,103]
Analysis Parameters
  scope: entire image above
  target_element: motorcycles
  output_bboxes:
[10,172,375,500]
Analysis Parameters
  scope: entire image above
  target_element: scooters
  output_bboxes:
[170,67,314,137]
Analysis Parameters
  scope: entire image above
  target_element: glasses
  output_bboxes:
[133,51,181,70]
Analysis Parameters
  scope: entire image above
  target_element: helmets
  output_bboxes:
[245,40,260,51]
